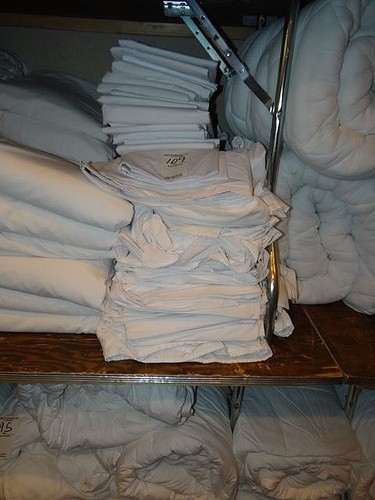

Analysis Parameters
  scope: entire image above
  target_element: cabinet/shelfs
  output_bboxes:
[0,0,374,429]
